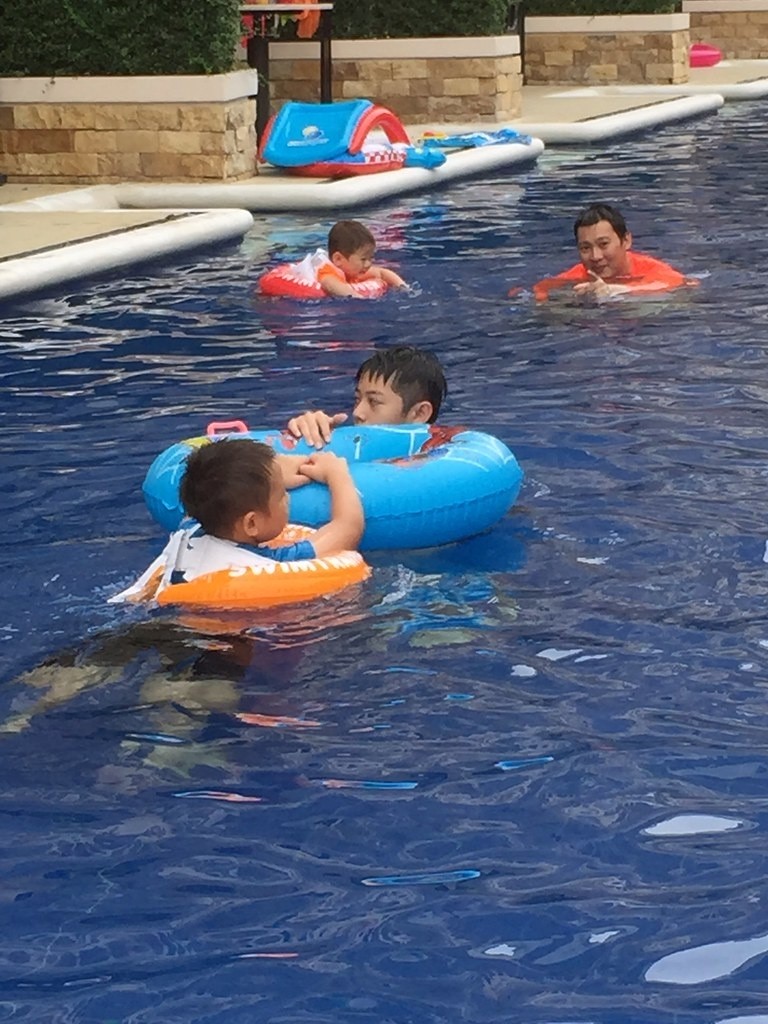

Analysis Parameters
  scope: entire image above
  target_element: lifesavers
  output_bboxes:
[143,423,523,550]
[690,44,722,67]
[107,525,365,609]
[285,140,405,176]
[256,263,388,300]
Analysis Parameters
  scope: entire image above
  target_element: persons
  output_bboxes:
[162,437,364,587]
[291,221,406,298]
[558,204,683,293]
[288,347,447,449]
